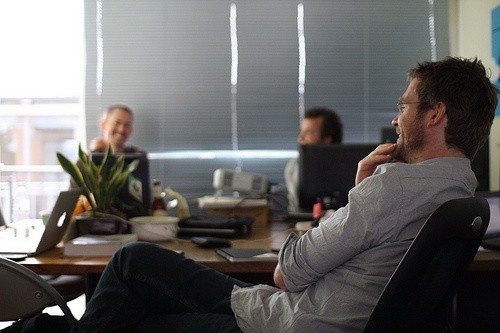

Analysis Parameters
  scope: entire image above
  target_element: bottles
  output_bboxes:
[149,180,167,216]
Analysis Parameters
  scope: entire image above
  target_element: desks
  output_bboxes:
[0,221,500,305]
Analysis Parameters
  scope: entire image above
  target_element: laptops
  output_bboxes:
[0,189,82,255]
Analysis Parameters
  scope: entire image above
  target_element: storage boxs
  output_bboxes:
[210,202,270,228]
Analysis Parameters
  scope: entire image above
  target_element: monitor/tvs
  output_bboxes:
[297,143,384,213]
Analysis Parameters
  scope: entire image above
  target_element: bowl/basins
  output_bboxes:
[129,216,180,242]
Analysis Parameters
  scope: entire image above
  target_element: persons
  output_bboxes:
[72,57,500,333]
[284,107,343,214]
[71,105,152,215]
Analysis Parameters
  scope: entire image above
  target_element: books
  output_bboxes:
[215,248,279,264]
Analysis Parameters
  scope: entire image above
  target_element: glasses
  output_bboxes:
[397,97,422,113]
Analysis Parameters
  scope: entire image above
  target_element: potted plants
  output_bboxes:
[56,142,139,236]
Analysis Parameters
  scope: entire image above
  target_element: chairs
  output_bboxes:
[366,198,489,333]
[0,257,76,333]
[39,272,85,307]
